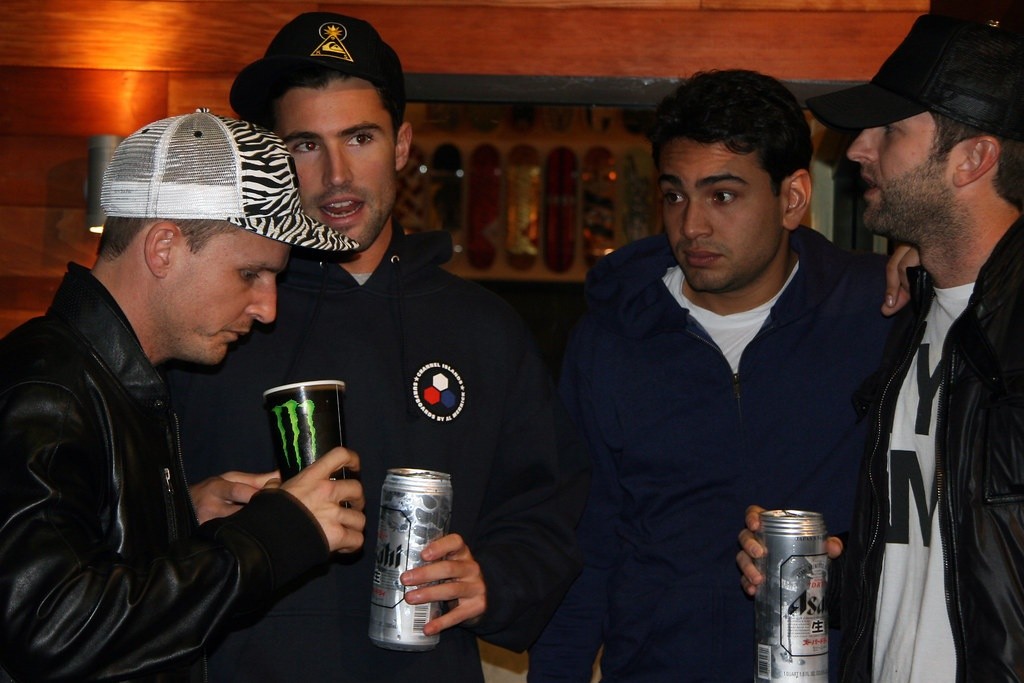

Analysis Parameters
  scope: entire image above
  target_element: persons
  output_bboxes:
[737,12,1024,683]
[0,108,368,682]
[527,70,921,683]
[163,12,586,683]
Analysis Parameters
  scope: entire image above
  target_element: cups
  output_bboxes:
[265,379,351,513]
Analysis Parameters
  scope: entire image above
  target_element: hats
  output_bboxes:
[100,108,361,251]
[229,11,407,129]
[804,14,1024,142]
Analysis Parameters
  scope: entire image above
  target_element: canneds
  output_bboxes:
[752,508,830,683]
[368,469,454,649]
